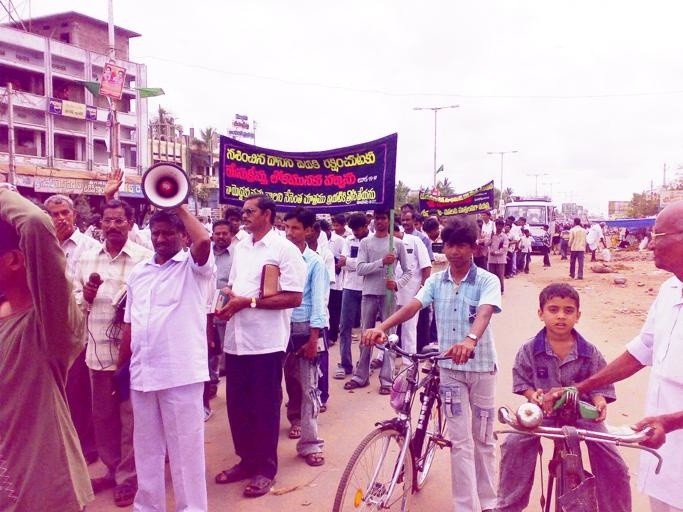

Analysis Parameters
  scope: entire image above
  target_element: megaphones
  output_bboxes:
[141,162,190,209]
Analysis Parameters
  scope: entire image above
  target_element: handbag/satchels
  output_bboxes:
[558,426,599,512]
[259,264,283,299]
[289,327,329,357]
[112,359,130,402]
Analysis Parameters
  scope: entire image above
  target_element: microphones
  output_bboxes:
[86,273,99,312]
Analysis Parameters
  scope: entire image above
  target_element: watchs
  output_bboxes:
[466,334,480,348]
[248,297,259,311]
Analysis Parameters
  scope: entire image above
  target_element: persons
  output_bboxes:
[213,192,304,500]
[69,198,156,507]
[0,188,96,511]
[102,65,111,82]
[359,214,502,511]
[541,198,683,512]
[112,71,124,84]
[0,164,652,469]
[117,202,212,511]
[493,282,632,512]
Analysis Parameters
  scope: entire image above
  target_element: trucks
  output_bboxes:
[502,195,562,254]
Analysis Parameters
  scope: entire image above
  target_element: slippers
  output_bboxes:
[204,406,212,422]
[244,475,276,496]
[216,465,251,483]
[320,403,326,412]
[333,359,392,394]
[289,425,301,438]
[305,453,325,466]
[114,486,135,507]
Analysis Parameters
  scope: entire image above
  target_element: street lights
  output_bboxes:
[412,105,461,187]
[526,173,548,197]
[557,190,574,201]
[543,182,560,195]
[487,151,520,198]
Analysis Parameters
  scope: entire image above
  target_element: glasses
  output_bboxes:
[651,228,683,240]
[240,208,255,216]
[101,218,129,227]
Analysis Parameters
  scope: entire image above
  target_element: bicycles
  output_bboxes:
[493,403,663,511]
[330,333,476,512]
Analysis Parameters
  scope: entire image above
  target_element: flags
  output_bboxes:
[218,129,396,215]
[137,88,166,98]
[77,81,98,98]
